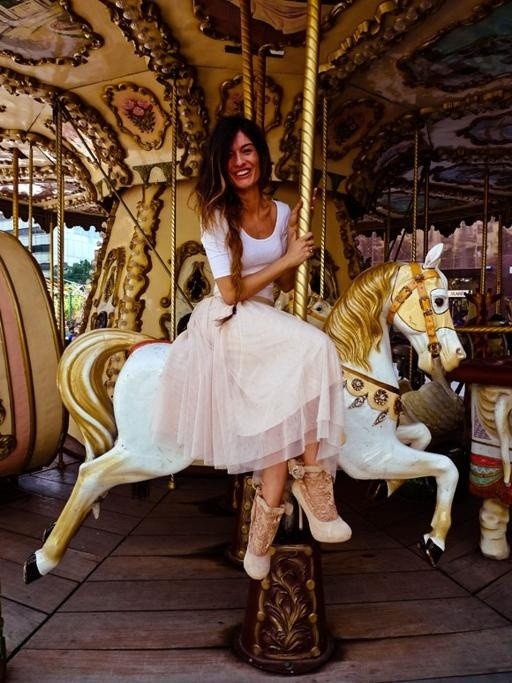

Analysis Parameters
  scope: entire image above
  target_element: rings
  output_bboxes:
[310,246,314,253]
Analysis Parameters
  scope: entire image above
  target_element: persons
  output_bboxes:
[156,116,353,581]
[64,327,76,341]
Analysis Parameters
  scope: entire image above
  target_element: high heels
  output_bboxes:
[242,481,293,580]
[286,458,352,543]
